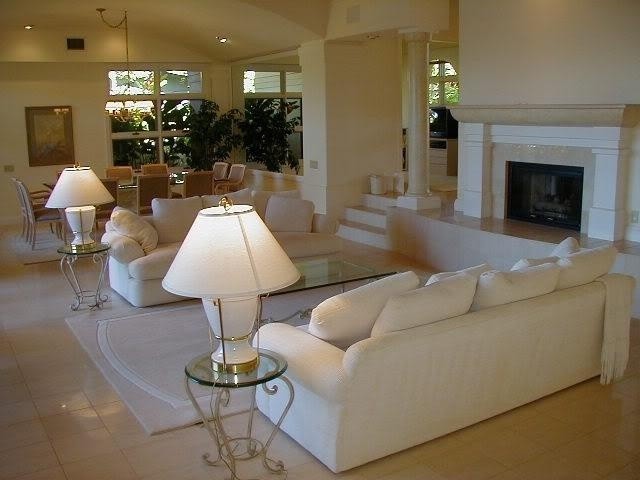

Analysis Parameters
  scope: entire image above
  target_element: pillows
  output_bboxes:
[111,188,312,252]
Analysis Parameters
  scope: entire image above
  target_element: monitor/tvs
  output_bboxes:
[507,161,584,231]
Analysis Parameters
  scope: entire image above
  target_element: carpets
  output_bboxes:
[7,231,70,265]
[64,268,440,437]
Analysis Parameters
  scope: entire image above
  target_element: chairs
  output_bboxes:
[102,164,214,217]
[209,160,247,195]
[10,174,70,248]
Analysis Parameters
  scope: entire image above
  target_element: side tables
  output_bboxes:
[184,345,293,479]
[57,245,111,312]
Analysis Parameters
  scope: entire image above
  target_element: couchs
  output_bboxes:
[102,187,342,308]
[252,274,634,475]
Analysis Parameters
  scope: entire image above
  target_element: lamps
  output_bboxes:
[46,164,115,247]
[160,199,300,377]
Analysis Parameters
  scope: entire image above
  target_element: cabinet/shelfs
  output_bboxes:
[428,148,448,177]
